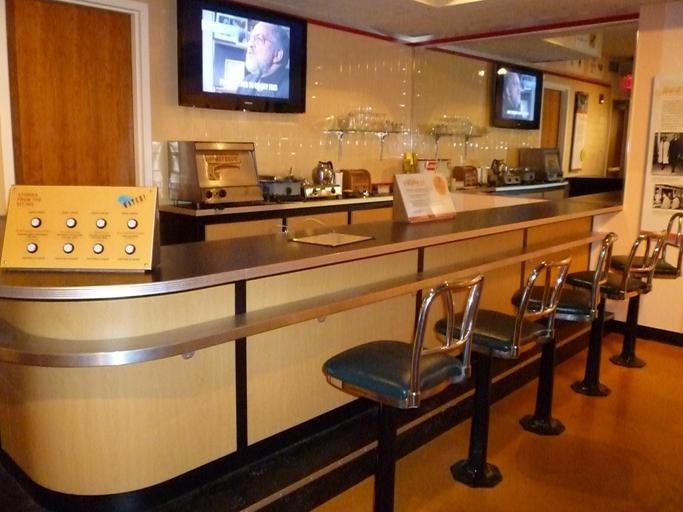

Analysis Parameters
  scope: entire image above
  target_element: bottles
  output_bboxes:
[403,152,417,173]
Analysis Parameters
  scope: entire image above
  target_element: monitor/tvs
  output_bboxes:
[177,0,307,114]
[494,61,543,130]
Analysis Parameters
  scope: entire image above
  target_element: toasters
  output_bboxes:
[452,165,479,187]
[341,169,372,196]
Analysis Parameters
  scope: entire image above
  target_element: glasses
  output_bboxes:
[249,34,275,45]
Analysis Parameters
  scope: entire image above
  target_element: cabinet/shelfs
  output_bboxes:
[160,201,394,242]
[484,186,570,201]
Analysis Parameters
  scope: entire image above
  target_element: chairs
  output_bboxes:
[433,254,572,488]
[511,232,615,437]
[609,213,683,368]
[567,233,664,397]
[321,274,484,512]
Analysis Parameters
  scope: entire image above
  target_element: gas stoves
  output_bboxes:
[499,175,521,184]
[301,181,342,199]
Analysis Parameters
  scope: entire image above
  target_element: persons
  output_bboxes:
[503,72,525,120]
[236,21,289,98]
[654,186,683,209]
[657,134,681,172]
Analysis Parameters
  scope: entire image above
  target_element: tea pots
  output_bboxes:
[491,160,507,176]
[312,159,335,183]
